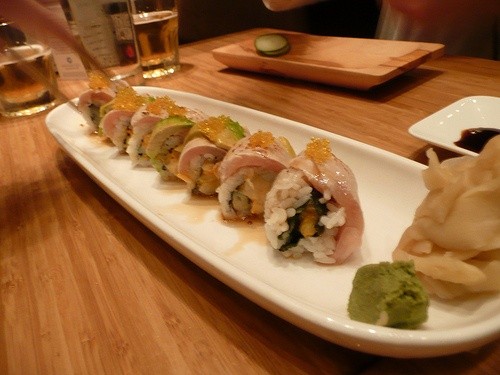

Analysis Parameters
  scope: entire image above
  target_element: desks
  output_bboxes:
[0,27,500,375]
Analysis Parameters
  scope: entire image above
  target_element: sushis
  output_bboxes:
[77,69,364,265]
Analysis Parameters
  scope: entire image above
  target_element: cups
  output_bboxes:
[0,15,61,120]
[58,0,144,93]
[127,0,181,82]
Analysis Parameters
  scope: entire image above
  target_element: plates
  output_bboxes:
[211,32,445,90]
[44,85,499,360]
[408,94,500,158]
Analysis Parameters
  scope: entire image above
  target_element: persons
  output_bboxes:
[259,0,500,65]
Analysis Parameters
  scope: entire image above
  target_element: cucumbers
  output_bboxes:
[255,34,289,57]
